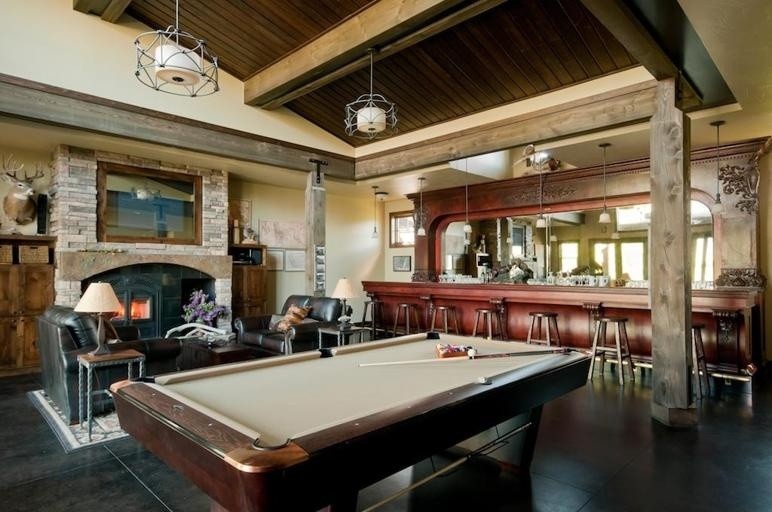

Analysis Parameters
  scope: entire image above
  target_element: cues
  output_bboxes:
[361,421,532,511]
[360,347,576,367]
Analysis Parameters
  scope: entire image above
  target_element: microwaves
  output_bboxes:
[464,253,490,268]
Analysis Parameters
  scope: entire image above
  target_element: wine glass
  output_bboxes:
[546,271,588,286]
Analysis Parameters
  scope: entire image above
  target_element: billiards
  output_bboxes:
[437,343,472,353]
[468,349,475,357]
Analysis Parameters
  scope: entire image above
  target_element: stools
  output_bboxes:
[363,302,561,347]
[588,318,635,386]
[691,324,710,399]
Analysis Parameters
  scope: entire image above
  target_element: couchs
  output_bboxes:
[37,307,182,424]
[233,296,343,357]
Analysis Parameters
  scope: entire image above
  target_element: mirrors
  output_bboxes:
[97,161,202,245]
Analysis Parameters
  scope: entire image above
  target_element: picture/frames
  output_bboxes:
[314,245,326,291]
[228,198,308,272]
[393,256,411,272]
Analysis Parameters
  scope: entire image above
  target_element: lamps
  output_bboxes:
[331,279,356,331]
[710,120,726,212]
[372,143,621,239]
[344,47,399,140]
[74,282,122,356]
[135,0,219,97]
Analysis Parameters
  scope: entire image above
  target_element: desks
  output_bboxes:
[109,333,592,512]
[76,349,145,443]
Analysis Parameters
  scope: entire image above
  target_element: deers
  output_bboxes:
[0,153,44,225]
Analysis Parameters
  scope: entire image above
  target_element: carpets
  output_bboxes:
[25,389,130,454]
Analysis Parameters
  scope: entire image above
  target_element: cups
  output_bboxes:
[438,273,492,284]
[609,280,616,288]
[624,280,648,288]
[527,279,535,285]
[692,281,714,289]
[597,276,608,287]
[587,276,597,287]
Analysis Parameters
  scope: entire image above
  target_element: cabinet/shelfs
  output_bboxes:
[229,245,268,316]
[0,236,57,377]
